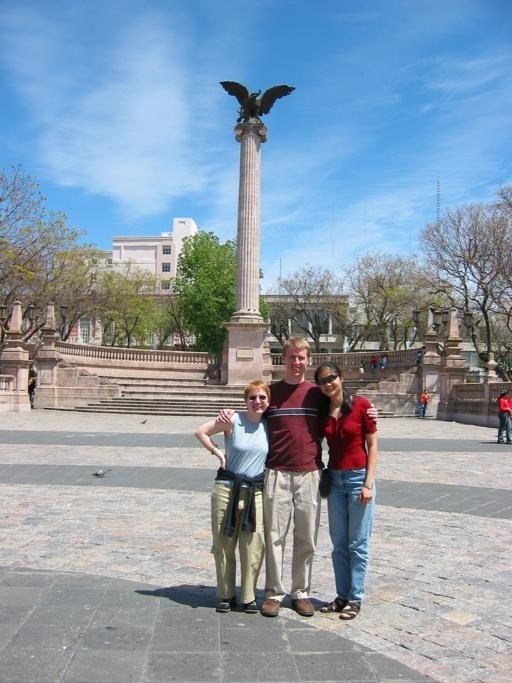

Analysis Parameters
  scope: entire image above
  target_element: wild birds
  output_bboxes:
[92,467,112,478]
[141,419,147,424]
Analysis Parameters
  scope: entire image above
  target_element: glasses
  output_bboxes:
[316,374,341,384]
[249,396,266,400]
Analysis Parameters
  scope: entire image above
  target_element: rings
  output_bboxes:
[366,500,368,503]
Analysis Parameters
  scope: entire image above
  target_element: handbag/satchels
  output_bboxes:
[320,469,330,498]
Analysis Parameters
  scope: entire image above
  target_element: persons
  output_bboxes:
[217,336,377,617]
[314,362,377,620]
[358,353,388,381]
[416,346,425,365]
[497,389,512,444]
[28,362,37,410]
[195,381,270,613]
[419,389,430,418]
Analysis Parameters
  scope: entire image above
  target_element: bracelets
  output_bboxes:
[364,483,372,490]
[211,445,219,455]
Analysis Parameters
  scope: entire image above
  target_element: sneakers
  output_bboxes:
[292,598,314,616]
[243,601,258,613]
[261,599,281,617]
[216,602,231,612]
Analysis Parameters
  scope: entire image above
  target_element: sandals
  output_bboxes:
[321,597,361,620]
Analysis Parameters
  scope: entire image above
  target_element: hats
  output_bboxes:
[500,390,509,394]
